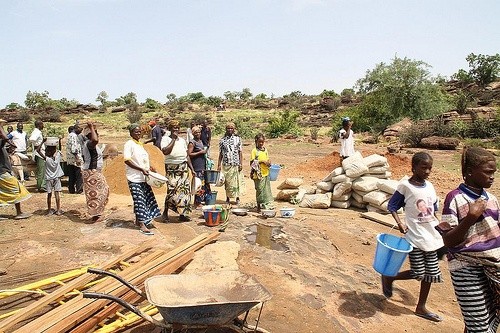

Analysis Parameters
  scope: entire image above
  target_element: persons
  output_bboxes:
[339,117,355,159]
[436,144,500,333]
[383,152,446,321]
[0,118,110,222]
[123,119,276,235]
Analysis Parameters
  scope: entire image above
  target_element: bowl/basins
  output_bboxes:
[201,205,214,215]
[145,171,169,189]
[215,172,225,187]
[191,176,202,196]
[280,208,296,218]
[42,137,60,146]
[261,210,277,218]
[232,209,249,216]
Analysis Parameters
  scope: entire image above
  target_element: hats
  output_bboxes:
[127,122,141,133]
[191,126,200,135]
[342,117,350,127]
[167,120,179,131]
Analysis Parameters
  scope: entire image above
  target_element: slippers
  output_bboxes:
[414,311,443,322]
[380,274,393,298]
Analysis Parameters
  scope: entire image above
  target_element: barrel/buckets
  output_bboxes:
[270,163,281,181]
[204,168,220,184]
[373,222,414,277]
[204,191,218,205]
[213,205,231,223]
[203,209,222,226]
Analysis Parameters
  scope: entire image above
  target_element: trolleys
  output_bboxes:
[83,266,273,333]
